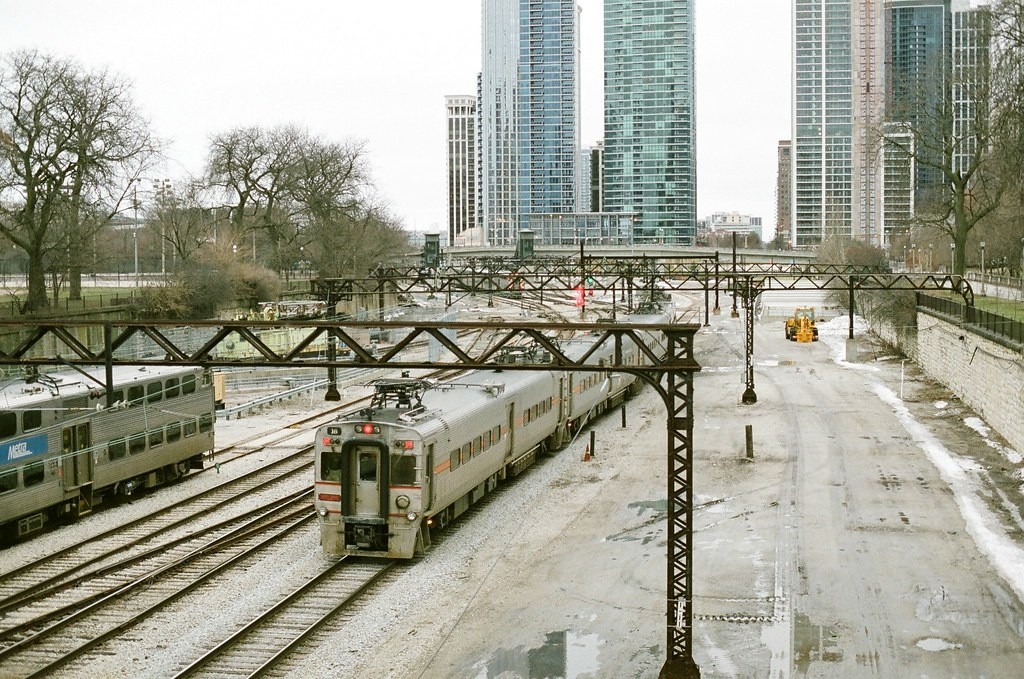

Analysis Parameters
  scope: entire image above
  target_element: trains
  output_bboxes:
[314,300,680,564]
[0,354,217,549]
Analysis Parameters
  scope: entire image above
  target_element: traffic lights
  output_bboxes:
[578,288,583,304]
[587,277,594,297]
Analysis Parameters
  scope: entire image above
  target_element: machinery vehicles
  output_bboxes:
[783,305,819,344]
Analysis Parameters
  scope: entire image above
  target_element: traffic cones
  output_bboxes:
[584,445,591,460]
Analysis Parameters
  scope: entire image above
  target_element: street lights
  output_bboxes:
[903,244,907,262]
[950,243,956,275]
[979,240,987,297]
[912,243,917,268]
[928,243,934,272]
[918,248,922,265]
